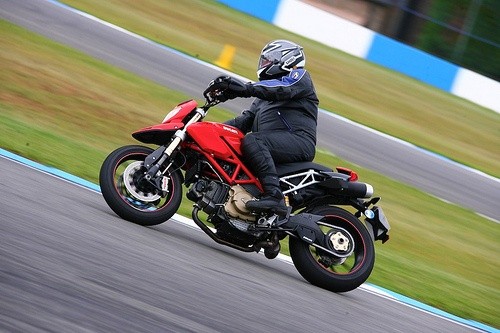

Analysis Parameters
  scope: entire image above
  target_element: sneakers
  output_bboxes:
[245,187,288,213]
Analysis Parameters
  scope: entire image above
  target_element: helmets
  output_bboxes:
[257,39,306,80]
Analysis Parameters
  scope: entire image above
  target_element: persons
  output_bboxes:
[208,40,319,214]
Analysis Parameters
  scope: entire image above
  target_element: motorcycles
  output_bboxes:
[98,73,391,294]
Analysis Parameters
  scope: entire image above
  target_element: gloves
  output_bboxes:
[215,74,255,98]
